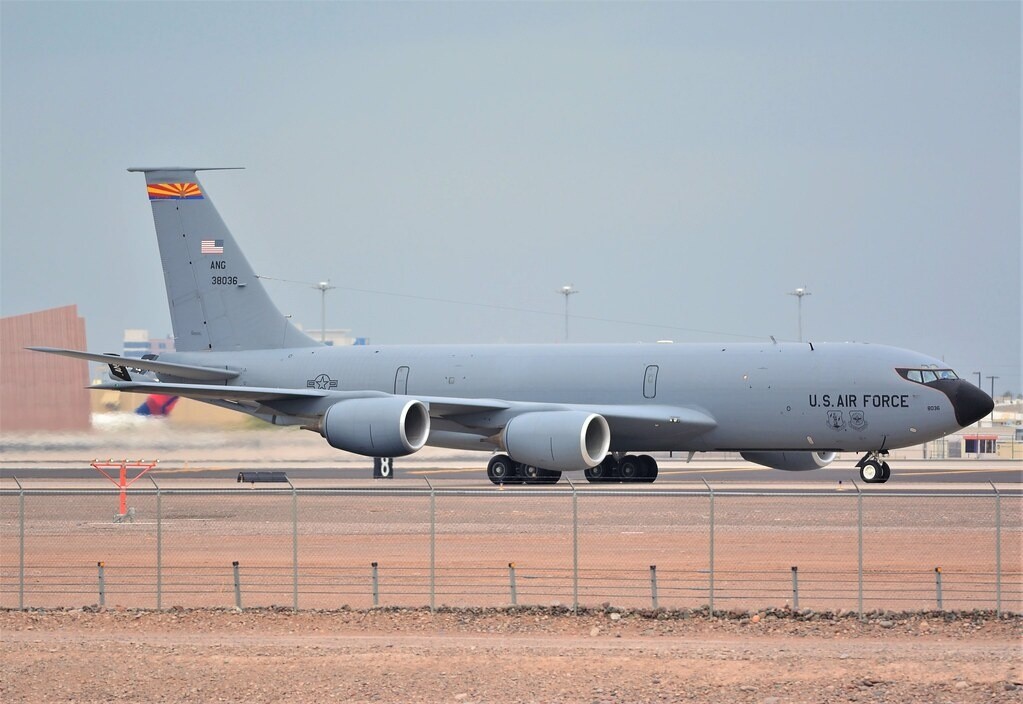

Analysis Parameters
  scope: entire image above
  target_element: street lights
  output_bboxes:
[313,282,334,340]
[788,287,813,342]
[556,287,579,342]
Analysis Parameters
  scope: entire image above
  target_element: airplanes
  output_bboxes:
[24,164,995,484]
